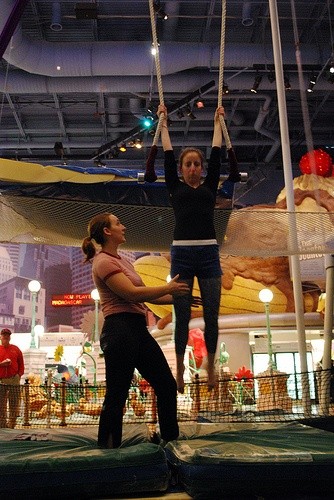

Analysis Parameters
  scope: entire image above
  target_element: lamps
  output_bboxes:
[153,0,168,20]
[329,60,334,73]
[284,72,291,88]
[307,70,317,93]
[177,96,204,120]
[268,72,275,83]
[222,85,229,94]
[250,65,262,93]
[97,127,156,166]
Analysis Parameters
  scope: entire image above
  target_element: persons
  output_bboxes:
[157,103,225,396]
[0,329,24,429]
[81,213,206,446]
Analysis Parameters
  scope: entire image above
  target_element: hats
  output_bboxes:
[1,329,12,335]
